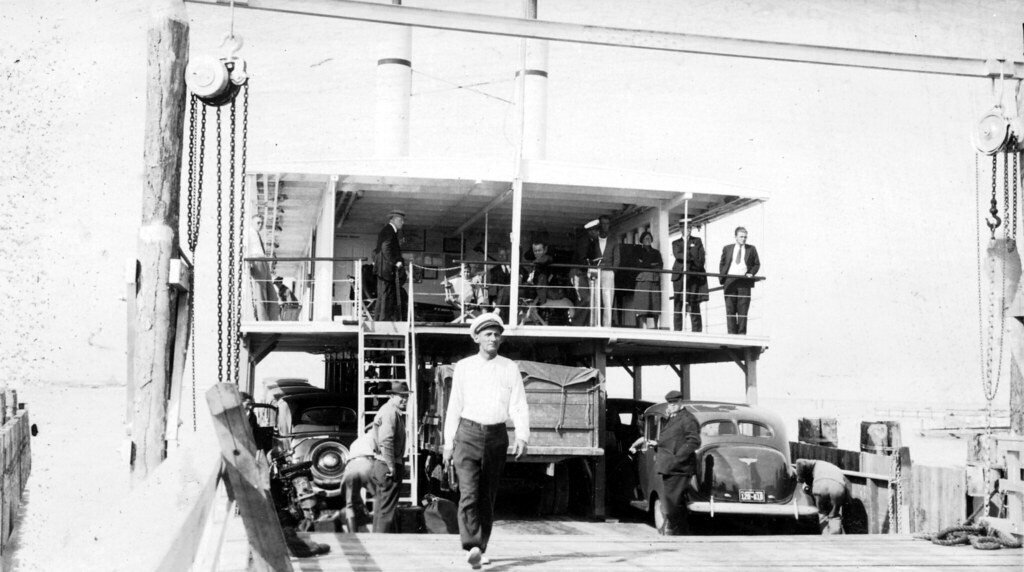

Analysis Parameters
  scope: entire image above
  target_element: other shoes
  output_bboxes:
[480,554,489,564]
[468,547,481,570]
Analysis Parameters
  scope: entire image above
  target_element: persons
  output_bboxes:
[245,214,281,322]
[792,458,850,536]
[525,242,574,306]
[586,214,620,326]
[369,209,409,321]
[654,392,701,534]
[563,227,599,321]
[369,381,413,531]
[440,315,531,570]
[339,427,377,535]
[451,259,494,321]
[718,227,761,335]
[240,394,328,558]
[274,277,300,310]
[630,232,662,328]
[487,246,524,319]
[671,216,710,332]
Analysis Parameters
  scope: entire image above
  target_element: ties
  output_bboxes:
[736,244,742,265]
[504,264,510,274]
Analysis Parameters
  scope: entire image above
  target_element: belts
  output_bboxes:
[461,418,505,432]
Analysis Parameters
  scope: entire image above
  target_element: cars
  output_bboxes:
[630,402,821,535]
[604,398,654,495]
[262,378,367,530]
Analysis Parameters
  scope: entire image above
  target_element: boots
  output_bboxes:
[346,517,358,532]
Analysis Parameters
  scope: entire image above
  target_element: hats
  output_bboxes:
[665,390,682,401]
[365,421,374,432]
[385,210,406,219]
[386,381,414,394]
[469,313,504,338]
[584,220,600,229]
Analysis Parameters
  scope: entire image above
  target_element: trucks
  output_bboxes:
[412,349,605,519]
